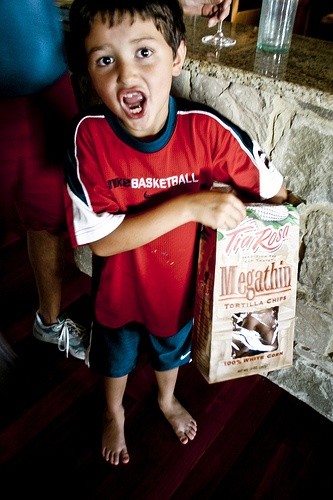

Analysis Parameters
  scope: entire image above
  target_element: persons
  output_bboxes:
[0,0,231,360]
[64,0,287,465]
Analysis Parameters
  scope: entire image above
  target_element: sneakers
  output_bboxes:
[33,309,85,360]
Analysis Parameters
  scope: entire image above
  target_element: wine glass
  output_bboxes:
[202,3,236,47]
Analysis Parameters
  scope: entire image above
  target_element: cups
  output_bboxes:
[257,0,299,53]
